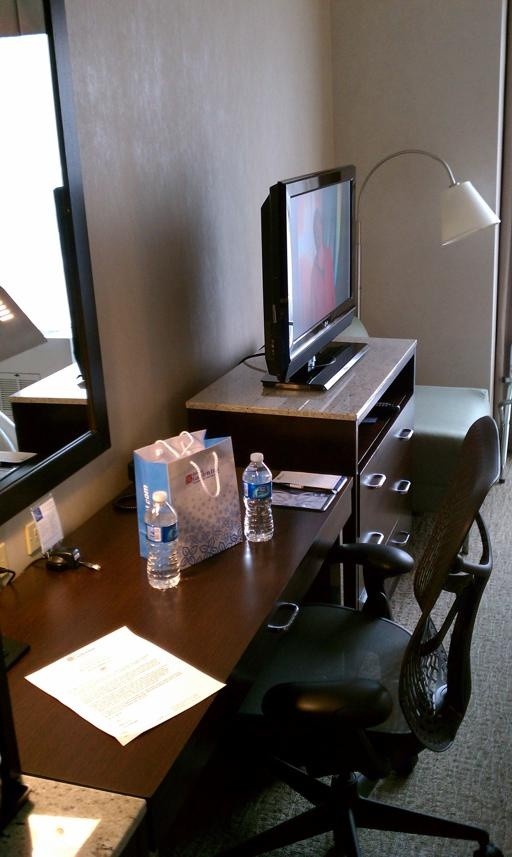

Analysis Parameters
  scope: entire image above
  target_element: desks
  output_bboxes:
[0,467,353,857]
[0,774,150,857]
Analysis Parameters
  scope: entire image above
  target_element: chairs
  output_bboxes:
[204,416,506,857]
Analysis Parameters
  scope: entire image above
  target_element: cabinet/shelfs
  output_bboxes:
[8,363,91,454]
[185,335,418,613]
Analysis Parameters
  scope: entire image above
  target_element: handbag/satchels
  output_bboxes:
[133,430,244,570]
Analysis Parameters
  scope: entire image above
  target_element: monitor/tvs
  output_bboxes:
[261,165,370,389]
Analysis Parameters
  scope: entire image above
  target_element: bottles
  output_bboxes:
[142,488,182,590]
[241,452,275,544]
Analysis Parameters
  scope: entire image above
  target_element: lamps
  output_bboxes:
[356,149,501,320]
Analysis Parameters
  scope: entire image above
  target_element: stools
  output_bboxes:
[413,384,489,555]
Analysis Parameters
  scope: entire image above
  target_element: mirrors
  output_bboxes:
[0,0,112,524]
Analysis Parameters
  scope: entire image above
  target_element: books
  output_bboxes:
[268,470,349,512]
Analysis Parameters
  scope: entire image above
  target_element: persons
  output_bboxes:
[306,206,343,323]
